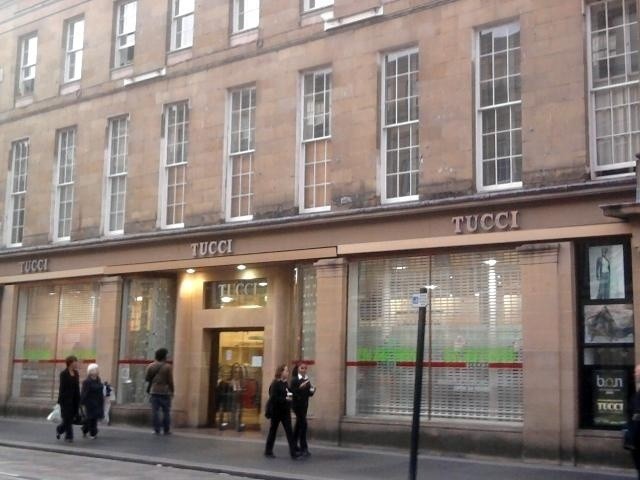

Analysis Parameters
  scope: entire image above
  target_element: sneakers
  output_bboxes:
[55,425,98,443]
[263,447,313,462]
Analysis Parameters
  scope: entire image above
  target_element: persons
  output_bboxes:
[626,364,640,477]
[594,245,610,301]
[263,363,308,462]
[54,354,81,445]
[289,360,316,457]
[78,361,105,441]
[144,347,175,436]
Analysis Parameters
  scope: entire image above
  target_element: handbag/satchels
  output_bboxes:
[264,399,279,420]
[46,400,89,424]
